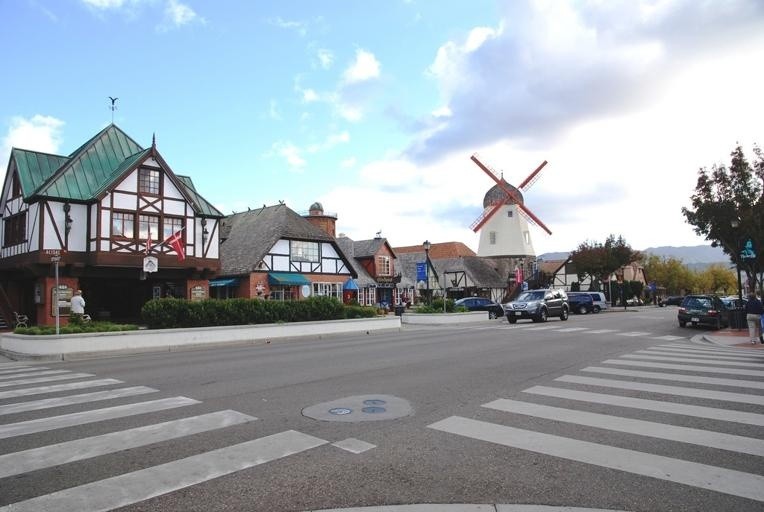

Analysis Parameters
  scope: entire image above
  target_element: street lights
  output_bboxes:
[422,240,431,306]
[729,216,742,300]
[201,215,209,258]
[63,199,73,251]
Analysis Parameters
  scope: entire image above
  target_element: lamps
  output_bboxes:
[65,216,73,233]
[203,229,209,240]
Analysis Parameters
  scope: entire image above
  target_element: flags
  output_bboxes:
[163,230,185,264]
[145,236,152,253]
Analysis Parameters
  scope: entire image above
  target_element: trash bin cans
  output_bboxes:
[82,315,91,323]
[489,306,497,319]
[395,307,405,316]
[729,309,745,329]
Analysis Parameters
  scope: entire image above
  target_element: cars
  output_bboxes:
[660,296,684,306]
[720,296,748,311]
[454,297,504,319]
[679,295,730,330]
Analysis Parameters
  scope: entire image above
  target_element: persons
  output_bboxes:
[70,289,85,320]
[744,291,762,344]
[756,290,764,343]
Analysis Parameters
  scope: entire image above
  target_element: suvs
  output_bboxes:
[566,291,607,314]
[505,289,569,324]
